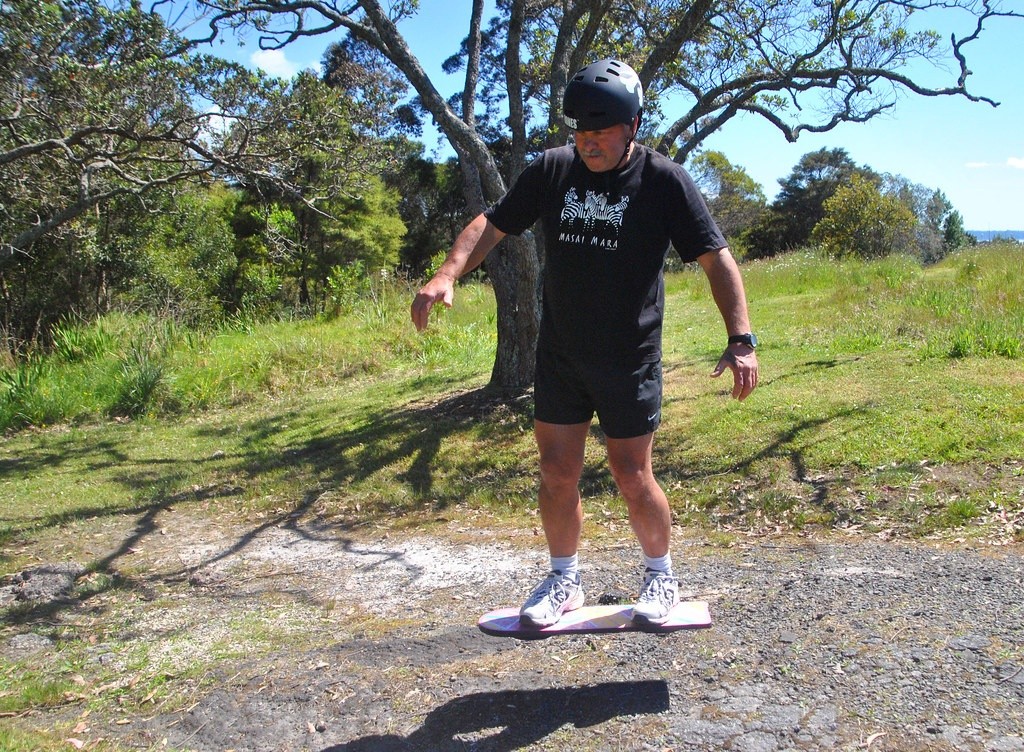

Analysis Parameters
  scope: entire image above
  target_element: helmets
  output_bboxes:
[562,59,644,129]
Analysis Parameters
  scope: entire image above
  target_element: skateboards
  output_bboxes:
[479,601,713,635]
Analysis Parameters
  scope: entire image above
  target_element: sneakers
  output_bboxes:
[517,568,586,630]
[630,570,680,626]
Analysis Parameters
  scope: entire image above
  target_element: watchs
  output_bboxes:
[728,333,757,349]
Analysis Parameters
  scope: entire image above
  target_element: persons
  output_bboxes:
[410,60,758,629]
